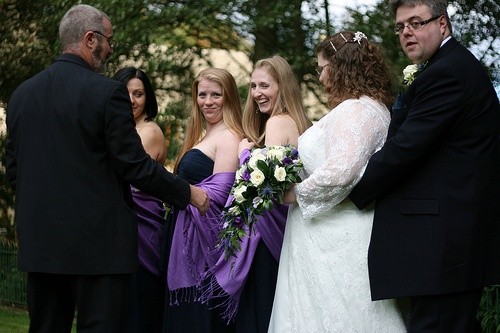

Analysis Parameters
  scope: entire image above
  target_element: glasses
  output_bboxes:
[315,63,329,74]
[394,14,440,34]
[94,31,117,49]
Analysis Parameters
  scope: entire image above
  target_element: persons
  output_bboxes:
[266,31,406,333]
[162,68,242,333]
[5,3,210,333]
[196,57,312,333]
[110,67,168,333]
[348,0,500,333]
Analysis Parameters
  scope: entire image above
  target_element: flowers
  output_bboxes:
[207,143,303,260]
[402,63,418,86]
